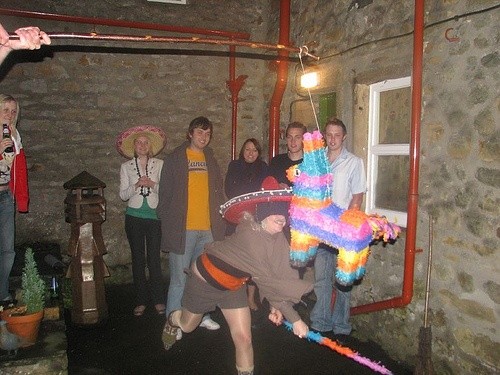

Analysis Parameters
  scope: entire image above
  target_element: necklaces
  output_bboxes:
[135,155,151,198]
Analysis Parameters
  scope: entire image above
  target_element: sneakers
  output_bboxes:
[1,297,18,306]
[133,304,167,316]
[176,313,220,340]
[310,329,349,345]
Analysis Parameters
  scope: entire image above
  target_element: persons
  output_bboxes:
[0,93,30,310]
[116,125,167,316]
[225,138,269,312]
[156,116,227,340]
[161,176,310,375]
[304,118,367,344]
[268,121,307,311]
[0,23,51,67]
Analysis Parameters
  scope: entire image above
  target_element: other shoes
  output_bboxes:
[162,310,178,350]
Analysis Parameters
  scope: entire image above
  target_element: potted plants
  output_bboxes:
[1,248,45,347]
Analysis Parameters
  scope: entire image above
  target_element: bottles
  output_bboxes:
[3,124,13,153]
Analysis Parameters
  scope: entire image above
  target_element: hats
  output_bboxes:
[220,176,294,225]
[116,125,167,159]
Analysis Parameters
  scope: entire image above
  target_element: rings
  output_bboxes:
[298,335,302,339]
[5,142,8,146]
